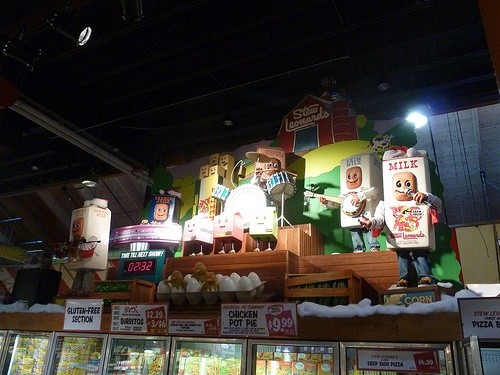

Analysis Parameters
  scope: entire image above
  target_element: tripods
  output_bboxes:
[272,190,294,230]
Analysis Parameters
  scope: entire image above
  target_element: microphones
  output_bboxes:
[404,188,437,210]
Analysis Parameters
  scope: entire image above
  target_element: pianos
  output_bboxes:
[110,223,182,248]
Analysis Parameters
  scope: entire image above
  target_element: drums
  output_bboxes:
[223,183,268,229]
[211,184,230,201]
[265,171,297,202]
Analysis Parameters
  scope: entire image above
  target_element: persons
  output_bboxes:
[65,199,112,293]
[358,145,442,287]
[253,146,286,207]
[320,152,384,253]
[141,189,182,225]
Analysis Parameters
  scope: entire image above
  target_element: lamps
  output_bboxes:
[0,35,47,71]
[43,13,93,48]
[223,113,235,130]
[119,0,143,24]
[81,167,101,188]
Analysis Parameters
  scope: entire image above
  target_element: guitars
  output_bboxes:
[304,190,366,217]
